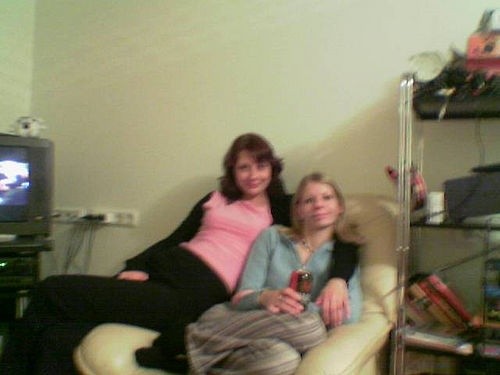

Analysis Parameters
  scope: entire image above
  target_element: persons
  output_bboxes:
[0,135,358,375]
[136,172,362,375]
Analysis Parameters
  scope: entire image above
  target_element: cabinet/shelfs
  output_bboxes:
[390,71,500,375]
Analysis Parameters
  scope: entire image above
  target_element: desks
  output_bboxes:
[0,240,55,353]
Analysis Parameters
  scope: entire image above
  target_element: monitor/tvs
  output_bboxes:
[0,133,54,235]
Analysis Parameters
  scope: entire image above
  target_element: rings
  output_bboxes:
[278,294,283,300]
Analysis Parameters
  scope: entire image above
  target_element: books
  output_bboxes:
[404,275,472,327]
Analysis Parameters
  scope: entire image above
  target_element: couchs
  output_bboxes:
[74,196,406,375]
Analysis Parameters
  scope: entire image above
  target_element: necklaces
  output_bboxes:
[298,234,313,254]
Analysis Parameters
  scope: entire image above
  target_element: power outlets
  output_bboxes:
[51,208,139,227]
